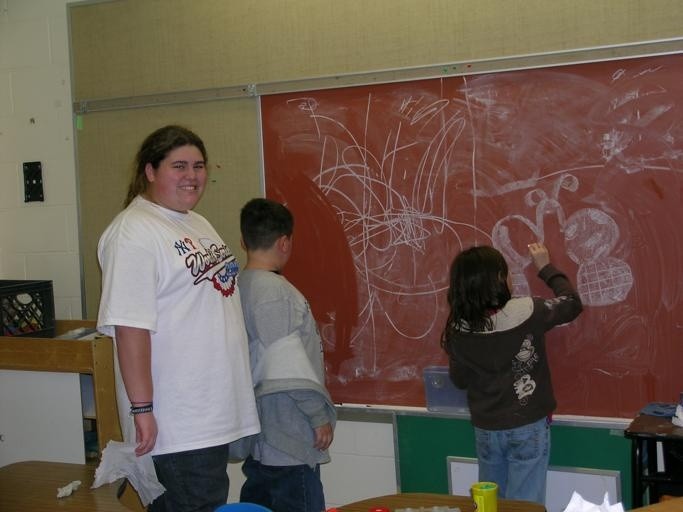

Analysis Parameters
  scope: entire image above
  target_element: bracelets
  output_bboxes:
[128,400,154,405]
[131,404,155,413]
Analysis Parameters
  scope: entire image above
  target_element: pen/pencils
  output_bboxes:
[117,479,127,499]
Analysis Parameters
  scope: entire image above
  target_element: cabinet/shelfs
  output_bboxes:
[1,320,122,464]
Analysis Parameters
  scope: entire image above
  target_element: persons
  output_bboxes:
[91,123,262,511]
[437,241,584,507]
[236,196,341,511]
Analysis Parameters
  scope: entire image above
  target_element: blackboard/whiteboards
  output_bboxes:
[392,412,648,512]
[256,40,683,430]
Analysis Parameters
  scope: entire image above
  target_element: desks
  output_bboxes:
[1,460,142,512]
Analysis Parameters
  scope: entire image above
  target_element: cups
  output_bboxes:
[470,481,499,512]
